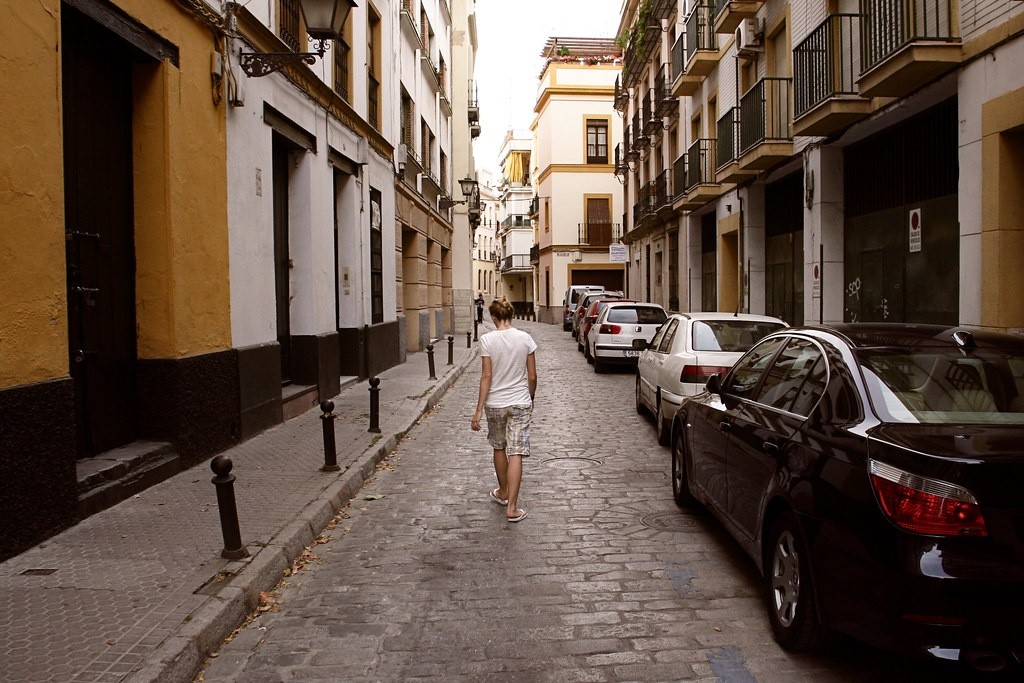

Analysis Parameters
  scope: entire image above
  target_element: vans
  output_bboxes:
[562,285,605,332]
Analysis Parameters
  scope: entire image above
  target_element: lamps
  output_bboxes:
[232,0,359,78]
[438,175,478,210]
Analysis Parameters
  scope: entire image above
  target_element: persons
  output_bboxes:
[475,293,484,323]
[471,296,537,521]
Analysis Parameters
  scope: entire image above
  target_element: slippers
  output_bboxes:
[489,488,509,505]
[508,508,528,521]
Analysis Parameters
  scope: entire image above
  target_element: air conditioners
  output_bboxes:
[735,17,759,51]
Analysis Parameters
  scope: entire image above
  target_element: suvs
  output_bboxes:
[569,290,625,343]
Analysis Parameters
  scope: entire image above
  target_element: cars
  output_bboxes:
[668,320,1024,674]
[574,298,642,358]
[631,311,800,449]
[584,301,669,374]
